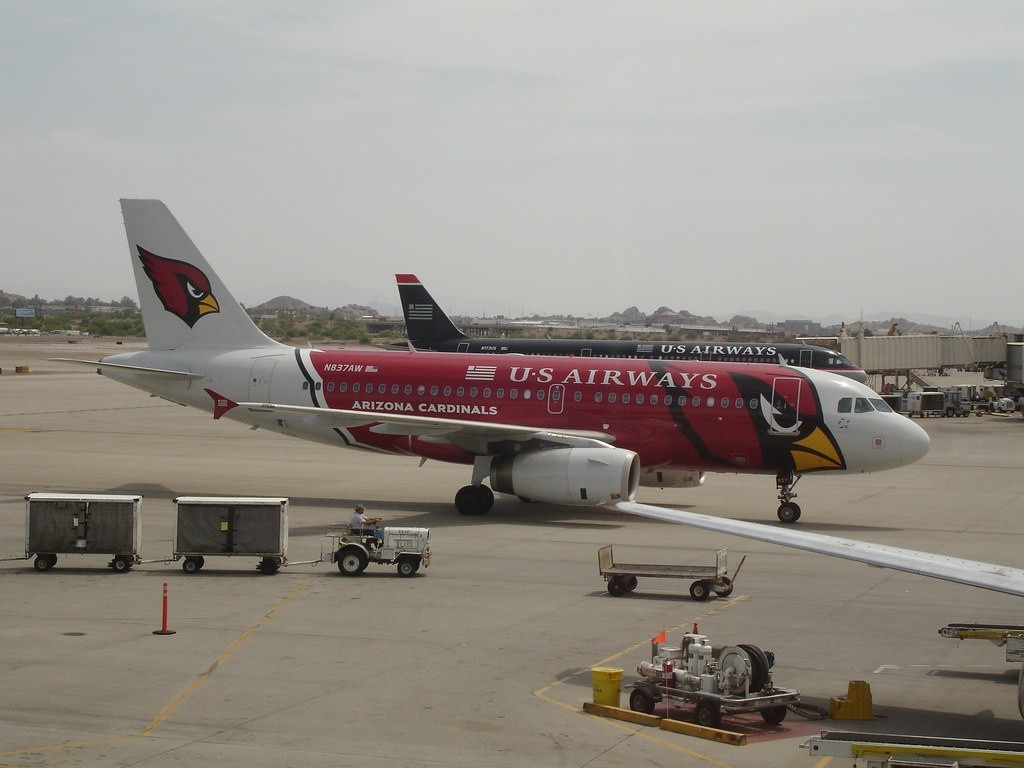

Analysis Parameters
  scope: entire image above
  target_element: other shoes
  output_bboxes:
[376,542,382,549]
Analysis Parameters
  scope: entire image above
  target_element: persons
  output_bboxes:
[885,383,891,395]
[348,504,382,543]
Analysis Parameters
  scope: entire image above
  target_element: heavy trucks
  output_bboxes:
[907,390,971,418]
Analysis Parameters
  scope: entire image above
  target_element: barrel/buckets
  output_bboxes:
[591,667,623,707]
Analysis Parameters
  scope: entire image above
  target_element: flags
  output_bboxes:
[653,631,666,645]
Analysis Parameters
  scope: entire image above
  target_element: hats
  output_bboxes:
[354,504,366,510]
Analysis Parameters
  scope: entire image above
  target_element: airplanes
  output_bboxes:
[39,198,933,520]
